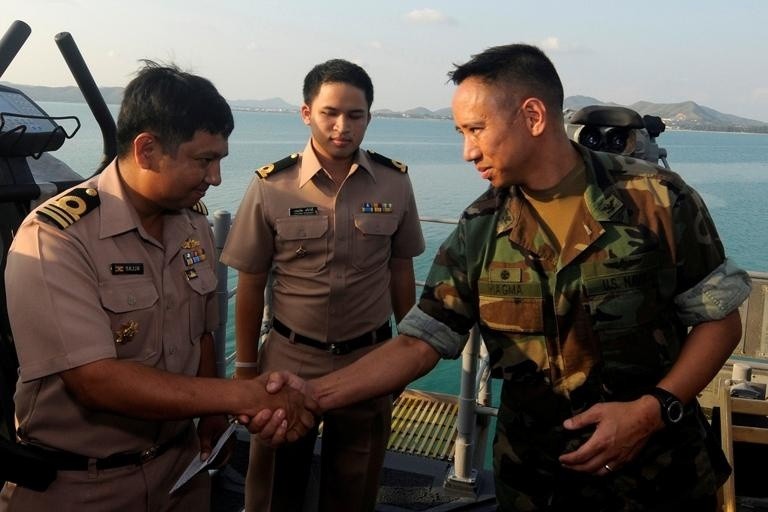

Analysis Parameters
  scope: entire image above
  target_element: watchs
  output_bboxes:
[644,386,683,424]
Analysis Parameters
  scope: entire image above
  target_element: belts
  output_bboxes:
[269,314,395,357]
[27,429,190,471]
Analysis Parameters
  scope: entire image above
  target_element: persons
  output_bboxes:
[220,59,427,510]
[1,55,320,511]
[236,43,754,509]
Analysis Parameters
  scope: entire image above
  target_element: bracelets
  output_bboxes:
[231,360,261,369]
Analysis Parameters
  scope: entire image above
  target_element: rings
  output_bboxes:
[605,464,613,472]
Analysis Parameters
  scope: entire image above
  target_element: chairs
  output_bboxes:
[714,376,767,512]
